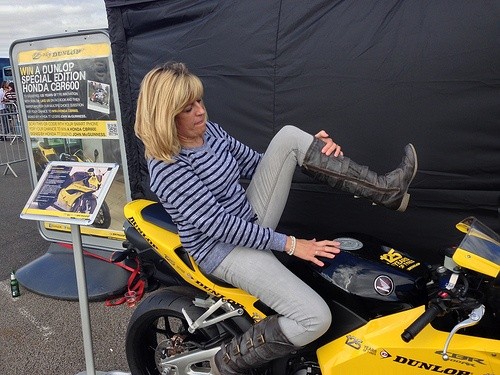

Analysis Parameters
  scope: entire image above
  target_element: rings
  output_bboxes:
[315,251,317,255]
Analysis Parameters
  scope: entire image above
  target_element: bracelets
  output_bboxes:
[286,236,296,255]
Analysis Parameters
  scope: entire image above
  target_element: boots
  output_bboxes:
[300,136,418,215]
[210,314,304,375]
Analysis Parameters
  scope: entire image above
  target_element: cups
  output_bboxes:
[125,289,137,307]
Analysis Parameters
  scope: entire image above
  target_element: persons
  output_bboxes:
[0,81,21,141]
[133,60,417,375]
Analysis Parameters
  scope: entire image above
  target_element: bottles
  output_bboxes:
[9,269,21,298]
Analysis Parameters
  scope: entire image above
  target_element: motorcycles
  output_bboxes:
[90,83,108,105]
[121,198,500,375]
[34,141,111,229]
[38,166,113,214]
